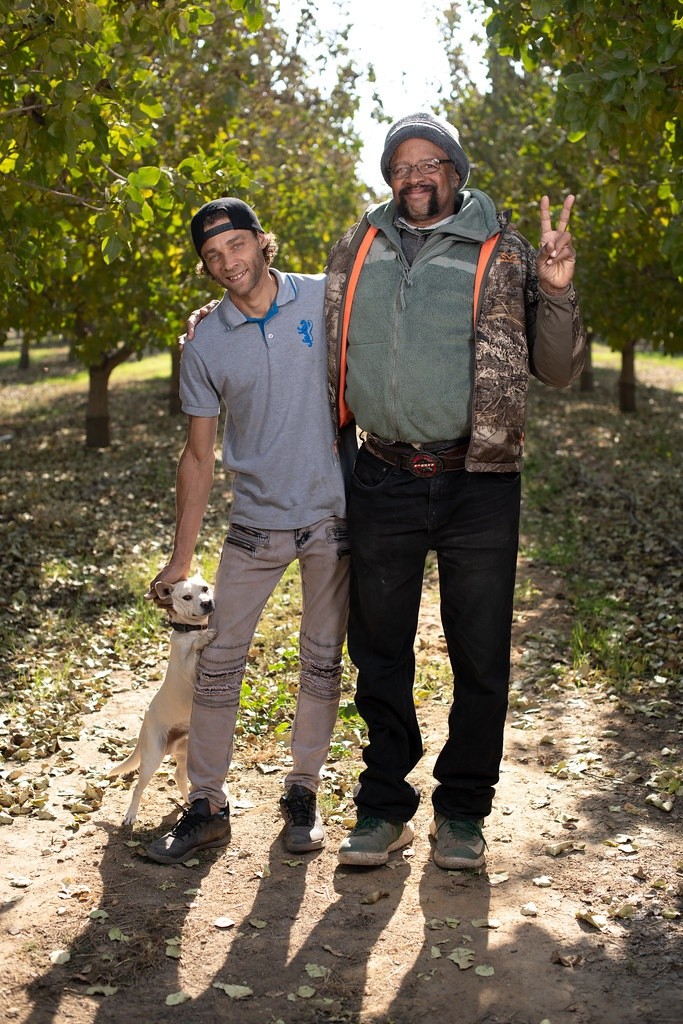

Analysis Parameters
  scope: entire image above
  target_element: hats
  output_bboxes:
[190,198,265,258]
[380,112,470,194]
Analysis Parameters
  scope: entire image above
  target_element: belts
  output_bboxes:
[363,430,466,479]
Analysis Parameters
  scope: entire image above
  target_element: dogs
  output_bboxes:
[106,568,219,826]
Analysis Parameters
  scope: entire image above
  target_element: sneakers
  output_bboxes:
[428,809,490,869]
[337,809,415,865]
[145,797,232,864]
[279,784,326,853]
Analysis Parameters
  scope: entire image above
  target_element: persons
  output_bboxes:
[188,114,586,869]
[146,198,350,865]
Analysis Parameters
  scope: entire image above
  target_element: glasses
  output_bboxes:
[387,158,453,180]
